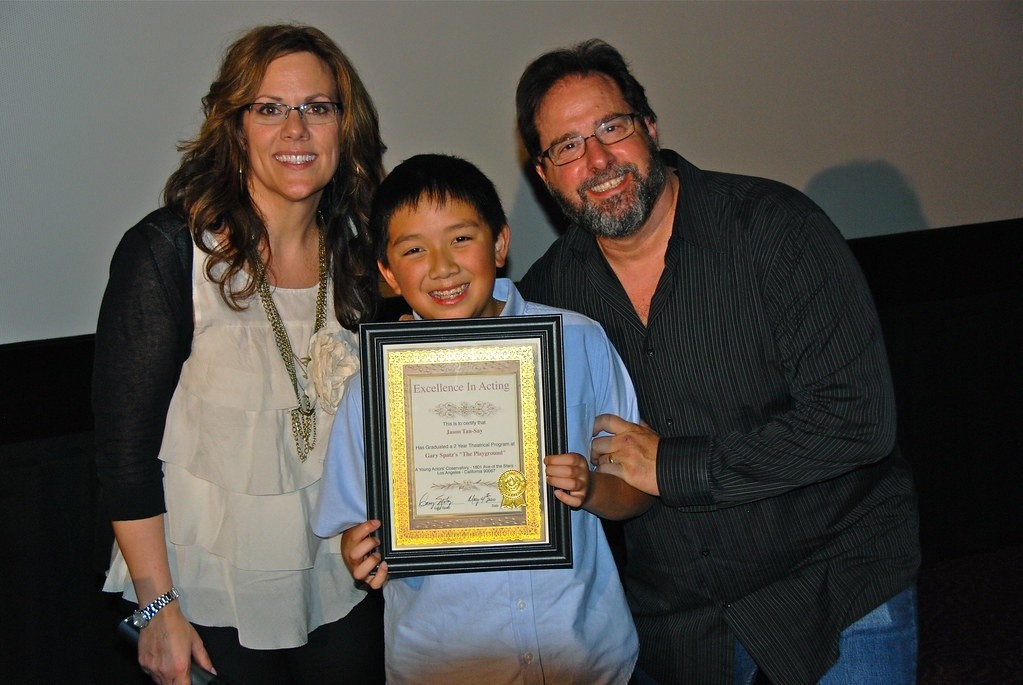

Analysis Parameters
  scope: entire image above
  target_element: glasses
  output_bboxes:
[537,110,643,167]
[245,101,344,125]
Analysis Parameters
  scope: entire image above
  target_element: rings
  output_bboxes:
[608,455,615,463]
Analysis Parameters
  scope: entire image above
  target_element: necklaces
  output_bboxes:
[219,187,342,466]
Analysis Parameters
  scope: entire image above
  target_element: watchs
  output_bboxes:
[132,579,179,631]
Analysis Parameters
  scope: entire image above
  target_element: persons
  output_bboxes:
[311,152,658,685]
[397,37,928,685]
[76,23,406,685]
